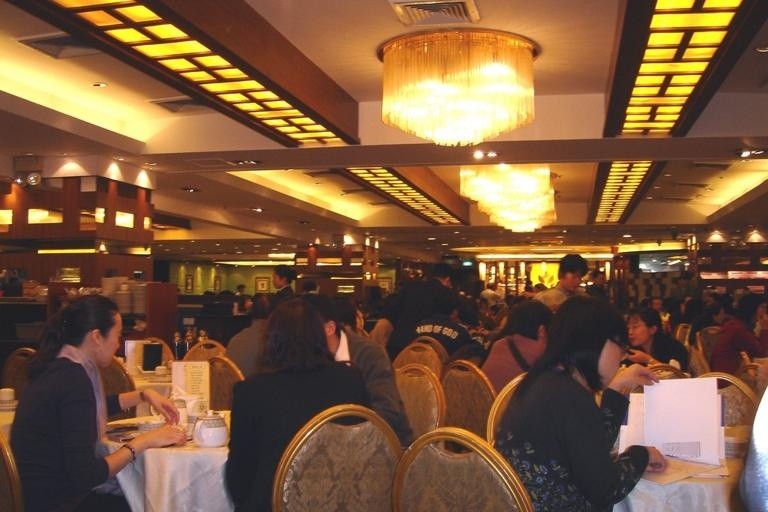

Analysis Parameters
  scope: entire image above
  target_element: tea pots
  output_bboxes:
[194,410,228,447]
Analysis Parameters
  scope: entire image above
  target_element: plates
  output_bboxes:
[137,365,161,373]
[150,375,170,382]
[1,404,20,411]
[102,276,147,316]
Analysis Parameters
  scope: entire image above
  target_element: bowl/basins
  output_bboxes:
[724,438,747,458]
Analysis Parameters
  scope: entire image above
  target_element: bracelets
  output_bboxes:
[121,444,137,462]
[141,388,146,402]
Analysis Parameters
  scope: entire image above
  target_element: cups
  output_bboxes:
[0,387,15,404]
[155,366,168,377]
[142,343,163,371]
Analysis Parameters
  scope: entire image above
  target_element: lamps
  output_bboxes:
[373,26,546,154]
[455,159,559,235]
[12,153,42,186]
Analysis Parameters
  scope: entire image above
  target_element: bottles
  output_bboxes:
[174,398,190,433]
[174,329,209,361]
[724,410,737,436]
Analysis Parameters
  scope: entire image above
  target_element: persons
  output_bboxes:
[10,293,187,511]
[222,252,768,512]
